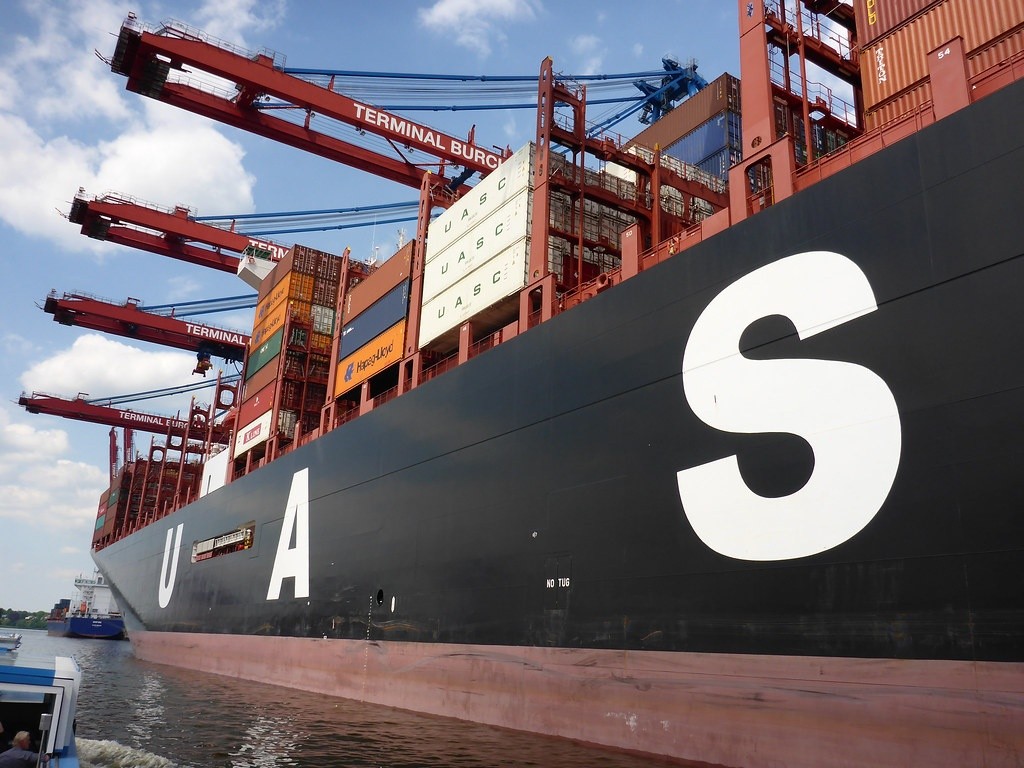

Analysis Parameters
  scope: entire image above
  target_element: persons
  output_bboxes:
[0,731,49,768]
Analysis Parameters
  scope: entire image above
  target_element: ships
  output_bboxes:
[13,1,1024,768]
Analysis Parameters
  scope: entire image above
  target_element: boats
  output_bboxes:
[1,652,81,768]
[0,631,24,654]
[43,561,127,639]
